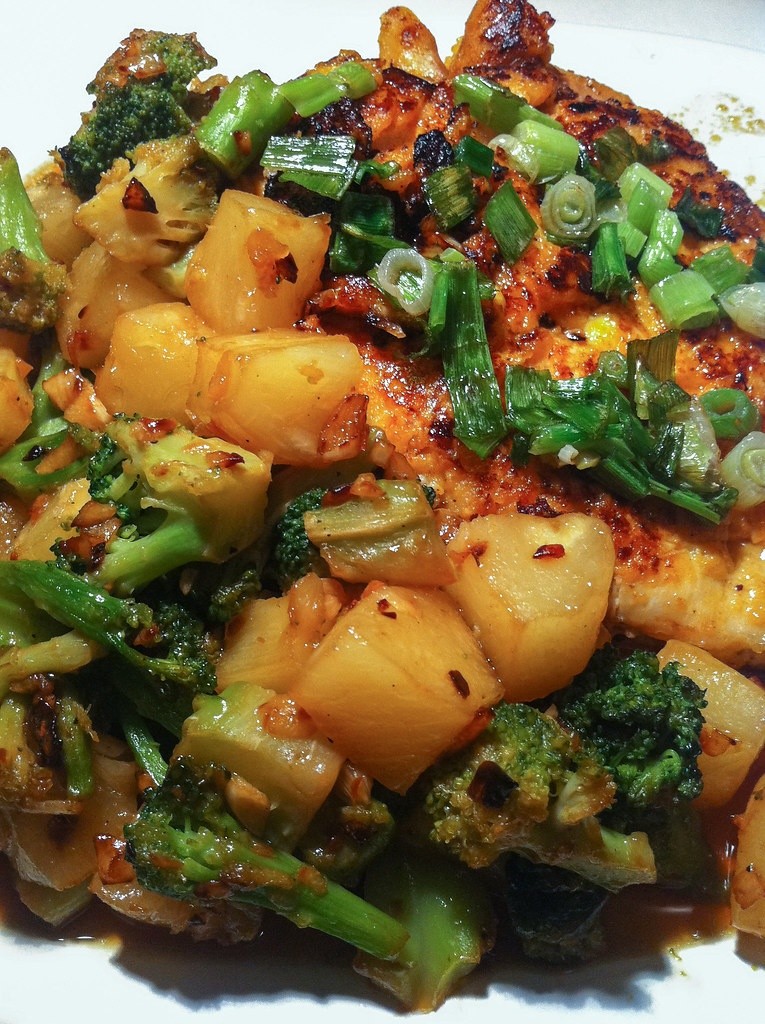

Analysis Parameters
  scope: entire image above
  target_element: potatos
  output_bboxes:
[3,164,765,942]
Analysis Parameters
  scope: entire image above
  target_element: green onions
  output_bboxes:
[0,56,765,516]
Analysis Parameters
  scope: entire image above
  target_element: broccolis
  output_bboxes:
[0,147,74,334]
[125,646,708,1014]
[0,559,220,702]
[269,486,335,589]
[50,28,216,201]
[48,411,270,597]
[71,135,224,265]
